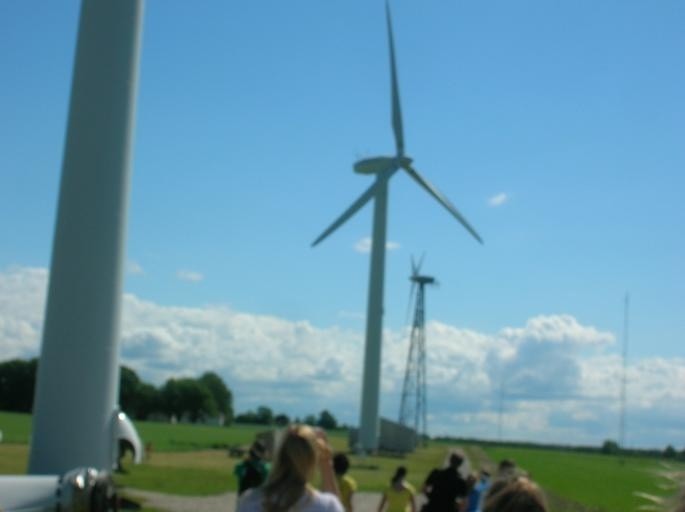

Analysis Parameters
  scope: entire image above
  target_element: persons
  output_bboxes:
[421,451,549,512]
[233,424,357,511]
[377,464,417,511]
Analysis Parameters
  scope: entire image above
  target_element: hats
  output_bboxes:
[451,451,464,463]
[237,439,267,458]
